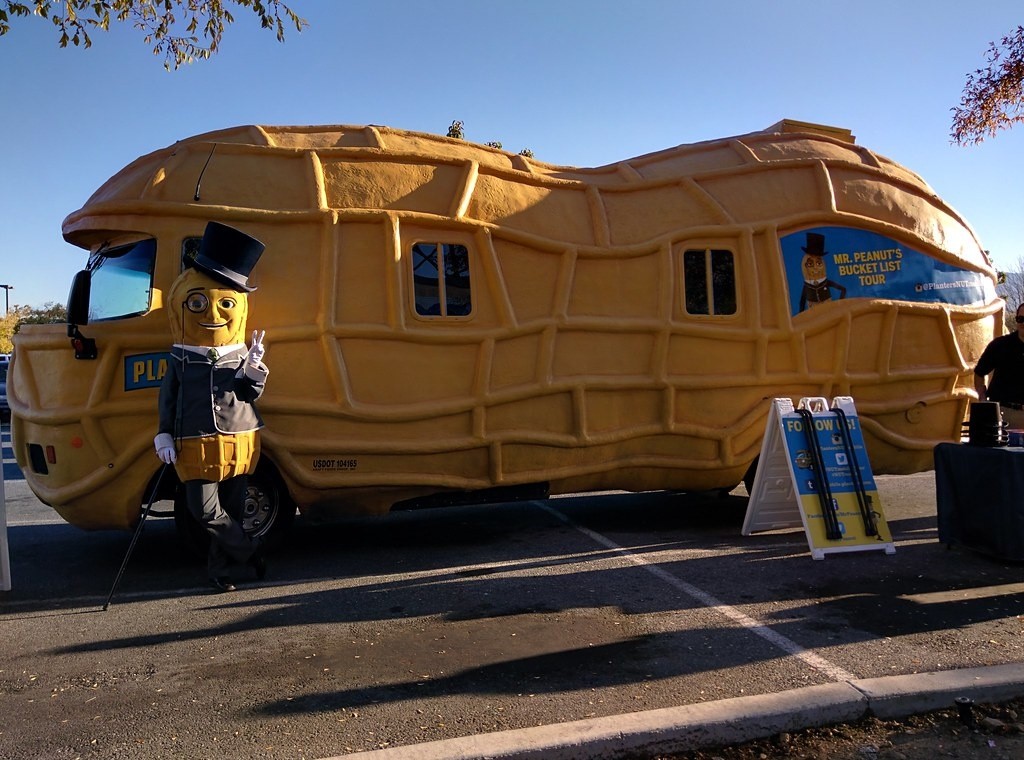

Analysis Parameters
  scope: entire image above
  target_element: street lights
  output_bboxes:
[0,285,14,316]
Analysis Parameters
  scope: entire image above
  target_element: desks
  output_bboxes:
[933,443,1024,565]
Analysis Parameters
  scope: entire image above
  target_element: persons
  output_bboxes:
[153,222,279,592]
[973,302,1024,429]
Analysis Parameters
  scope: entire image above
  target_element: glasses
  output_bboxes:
[1015,316,1024,323]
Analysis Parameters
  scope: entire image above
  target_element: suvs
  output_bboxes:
[0,354,14,412]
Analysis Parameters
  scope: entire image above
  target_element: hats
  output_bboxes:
[960,401,1010,447]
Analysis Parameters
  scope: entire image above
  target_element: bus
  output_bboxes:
[5,119,1010,564]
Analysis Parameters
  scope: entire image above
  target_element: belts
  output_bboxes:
[990,399,1024,410]
